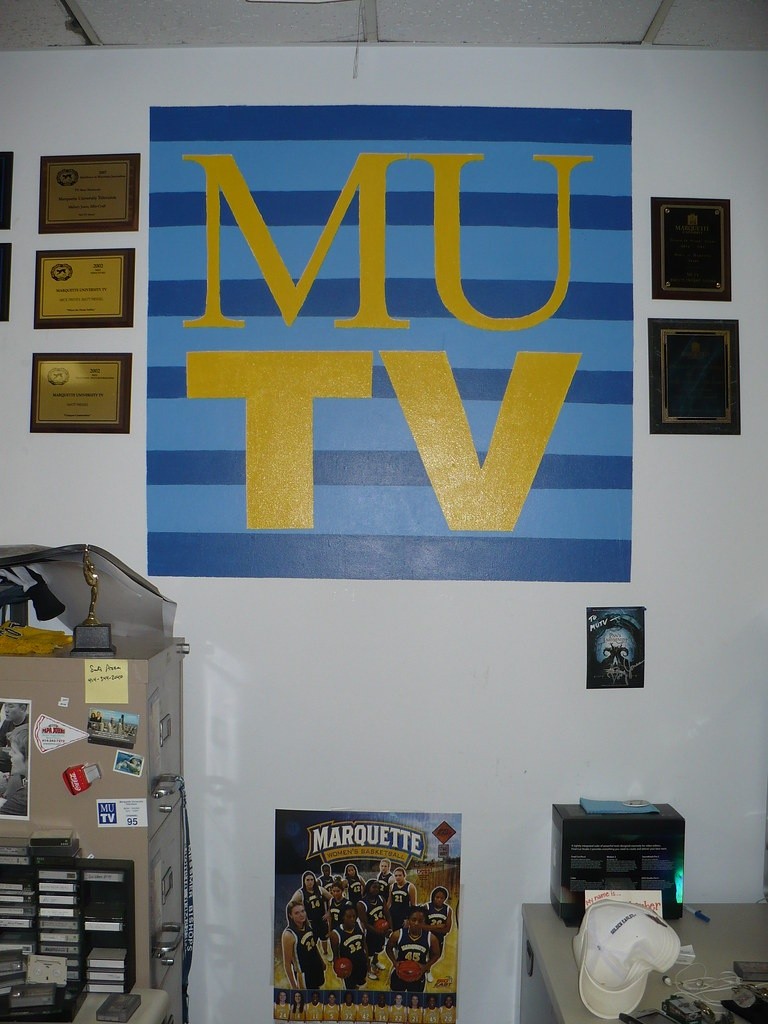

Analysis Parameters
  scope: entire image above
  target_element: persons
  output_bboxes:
[124,757,142,775]
[274,858,457,1024]
[0,702,29,817]
[90,711,102,722]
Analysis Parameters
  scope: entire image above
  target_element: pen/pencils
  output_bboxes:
[682,904,710,922]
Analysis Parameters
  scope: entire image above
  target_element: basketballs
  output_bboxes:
[334,958,352,977]
[374,919,390,932]
[398,960,422,983]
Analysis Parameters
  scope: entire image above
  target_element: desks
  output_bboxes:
[517,901,768,1024]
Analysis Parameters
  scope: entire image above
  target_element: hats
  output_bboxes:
[573,898,680,1019]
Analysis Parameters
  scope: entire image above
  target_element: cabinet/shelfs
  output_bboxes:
[0,629,187,1024]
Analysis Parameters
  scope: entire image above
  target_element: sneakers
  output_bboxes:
[368,972,377,980]
[375,962,386,969]
[425,972,433,982]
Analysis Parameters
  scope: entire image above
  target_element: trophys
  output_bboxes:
[70,542,117,657]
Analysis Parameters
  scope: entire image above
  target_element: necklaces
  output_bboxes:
[13,717,25,725]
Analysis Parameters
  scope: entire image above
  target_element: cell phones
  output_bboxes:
[630,1008,681,1024]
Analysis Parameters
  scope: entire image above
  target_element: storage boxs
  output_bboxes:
[549,804,687,928]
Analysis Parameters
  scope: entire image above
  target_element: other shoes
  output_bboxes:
[324,952,333,961]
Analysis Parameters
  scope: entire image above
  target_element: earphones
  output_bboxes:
[696,979,712,987]
[662,975,672,986]
